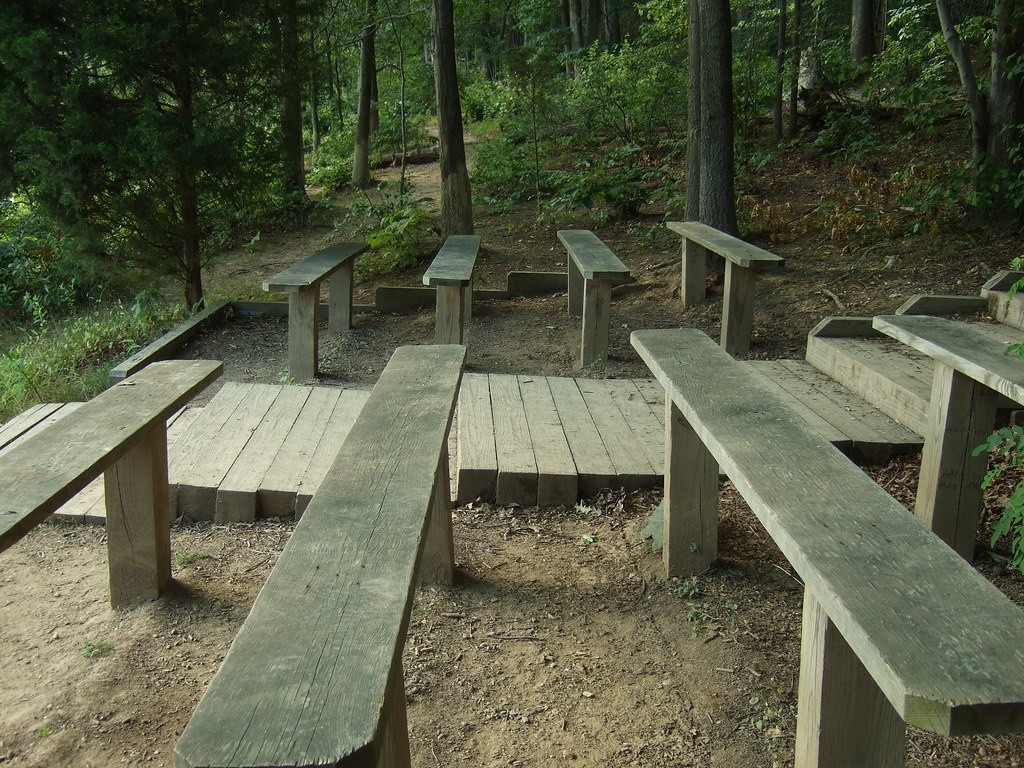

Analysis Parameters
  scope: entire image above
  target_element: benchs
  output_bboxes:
[175,343,469,768]
[422,236,482,344]
[666,221,784,355]
[631,328,1024,768]
[556,231,630,365]
[0,360,223,604]
[873,314,1024,564]
[263,242,372,380]
